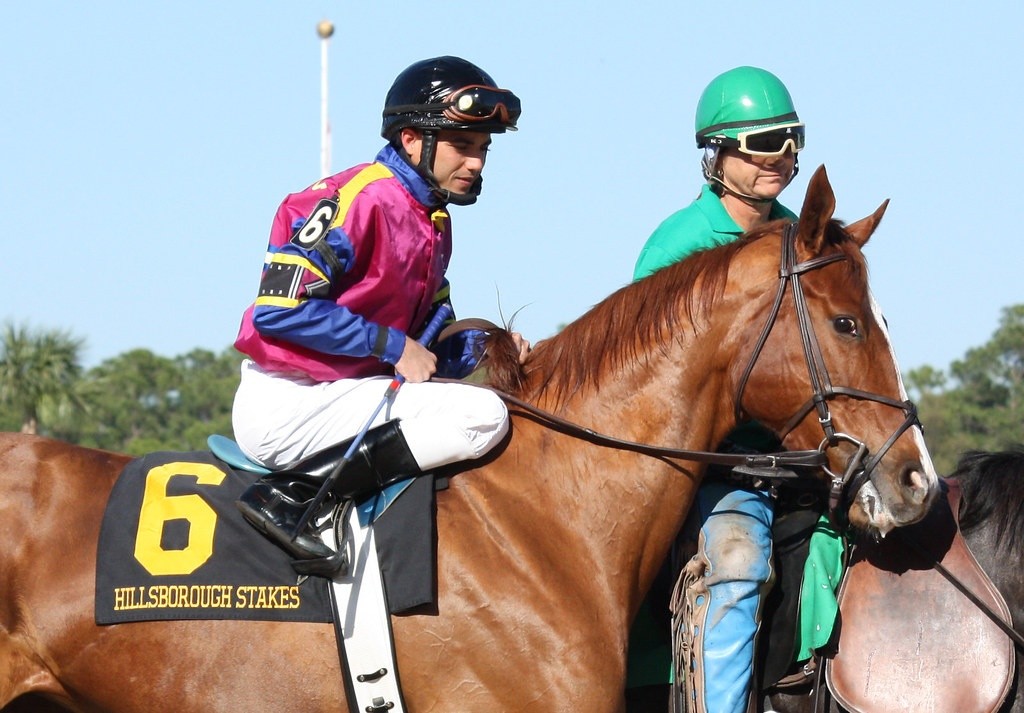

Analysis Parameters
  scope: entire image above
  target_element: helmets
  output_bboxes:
[380,54,523,141]
[694,66,805,148]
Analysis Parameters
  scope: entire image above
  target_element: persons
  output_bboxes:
[232,56,533,580]
[633,66,806,713]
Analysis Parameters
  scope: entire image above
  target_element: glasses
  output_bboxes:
[700,122,805,156]
[383,85,520,124]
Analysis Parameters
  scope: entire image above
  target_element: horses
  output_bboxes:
[0,163,940,713]
[625,451,1023,712]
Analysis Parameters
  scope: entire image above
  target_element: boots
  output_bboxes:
[238,419,424,559]
